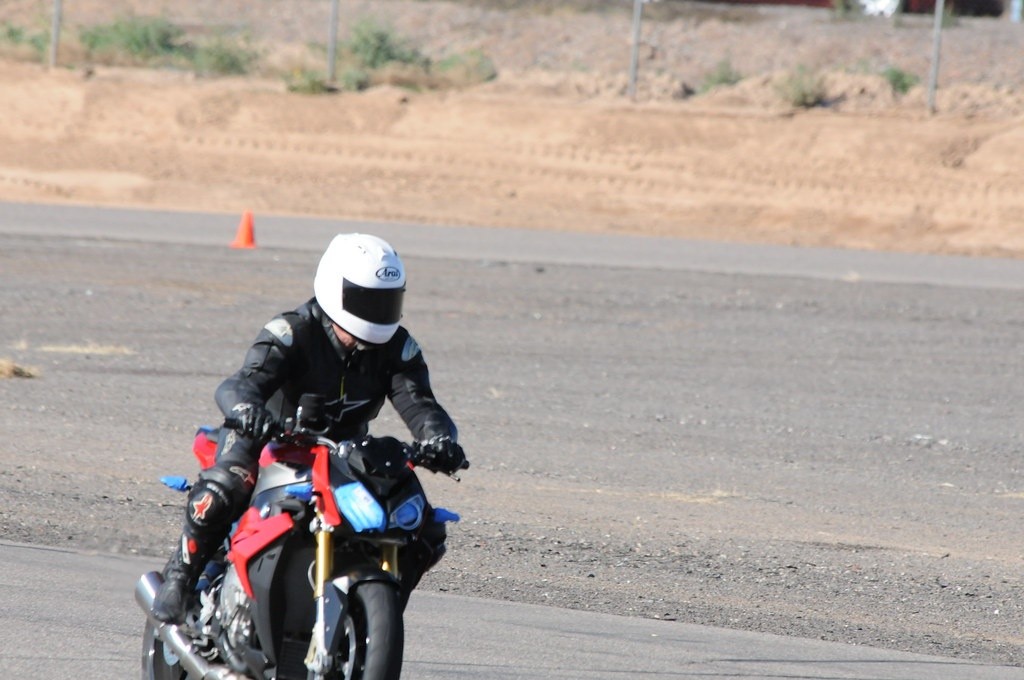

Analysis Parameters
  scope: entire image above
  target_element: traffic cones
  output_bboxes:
[226,210,257,250]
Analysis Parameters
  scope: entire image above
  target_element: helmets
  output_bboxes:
[313,233,407,345]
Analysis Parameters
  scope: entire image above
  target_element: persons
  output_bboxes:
[151,234,465,623]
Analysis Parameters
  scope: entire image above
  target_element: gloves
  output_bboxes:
[416,433,466,477]
[232,402,276,442]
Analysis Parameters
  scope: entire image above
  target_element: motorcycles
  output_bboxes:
[135,425,470,680]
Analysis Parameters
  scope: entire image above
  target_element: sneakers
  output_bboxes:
[150,545,202,626]
